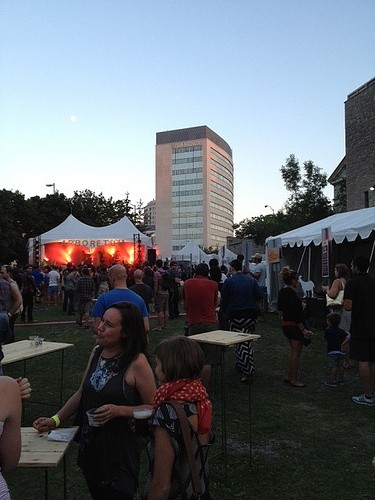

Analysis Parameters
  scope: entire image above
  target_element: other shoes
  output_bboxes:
[286,377,307,387]
[152,326,162,330]
[338,377,345,386]
[324,378,338,387]
[28,319,38,323]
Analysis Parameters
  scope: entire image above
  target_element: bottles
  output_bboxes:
[302,328,310,336]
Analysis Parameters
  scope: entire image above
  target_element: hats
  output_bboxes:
[209,259,219,266]
[251,253,262,261]
[67,262,72,266]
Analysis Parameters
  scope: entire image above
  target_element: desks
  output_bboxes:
[19,426,79,500]
[187,330,261,485]
[0,339,75,425]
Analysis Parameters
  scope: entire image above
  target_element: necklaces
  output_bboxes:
[93,349,116,392]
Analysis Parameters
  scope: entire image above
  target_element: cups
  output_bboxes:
[29,335,44,351]
[86,408,104,427]
[133,407,153,434]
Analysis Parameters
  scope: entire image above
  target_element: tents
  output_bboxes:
[265,206,375,313]
[207,245,238,266]
[28,214,151,266]
[160,239,211,270]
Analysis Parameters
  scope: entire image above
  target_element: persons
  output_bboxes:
[33,301,158,500]
[184,264,218,386]
[140,336,214,500]
[0,250,119,352]
[125,259,233,332]
[321,264,350,368]
[92,265,149,334]
[0,345,22,500]
[221,260,261,382]
[323,313,350,386]
[344,256,375,406]
[237,253,267,322]
[278,267,310,386]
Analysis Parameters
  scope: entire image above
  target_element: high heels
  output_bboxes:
[239,370,255,385]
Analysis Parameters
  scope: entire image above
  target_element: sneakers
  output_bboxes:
[352,392,375,406]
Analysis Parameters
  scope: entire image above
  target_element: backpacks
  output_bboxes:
[160,268,175,291]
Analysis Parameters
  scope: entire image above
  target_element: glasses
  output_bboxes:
[0,271,4,274]
[28,268,32,269]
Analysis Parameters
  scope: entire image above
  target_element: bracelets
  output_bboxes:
[51,415,60,427]
[8,312,12,317]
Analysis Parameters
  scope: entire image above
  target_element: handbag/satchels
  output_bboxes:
[64,284,73,292]
[325,279,345,306]
